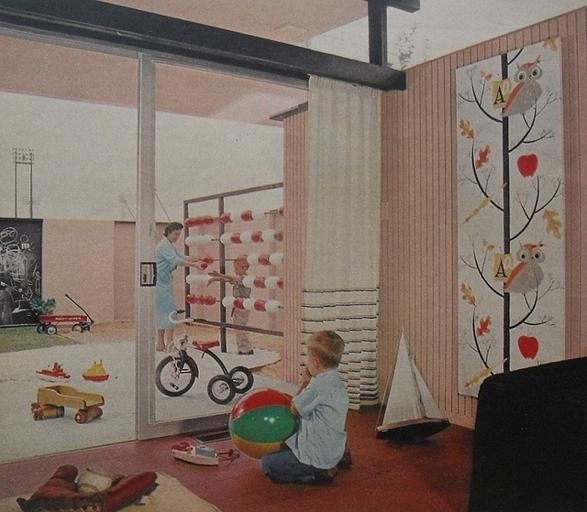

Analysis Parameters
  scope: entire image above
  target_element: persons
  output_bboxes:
[154,223,208,351]
[262,330,351,485]
[207,258,253,355]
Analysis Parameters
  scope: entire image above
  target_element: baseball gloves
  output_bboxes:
[17,464,159,511]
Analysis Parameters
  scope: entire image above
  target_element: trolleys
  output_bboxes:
[37,294,95,335]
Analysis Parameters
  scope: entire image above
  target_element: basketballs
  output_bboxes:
[229,387,300,459]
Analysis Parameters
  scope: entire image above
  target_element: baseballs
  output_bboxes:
[75,467,111,494]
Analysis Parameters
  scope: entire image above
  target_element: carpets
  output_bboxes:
[1,471,222,512]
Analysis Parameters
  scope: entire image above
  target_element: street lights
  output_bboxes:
[12,145,34,220]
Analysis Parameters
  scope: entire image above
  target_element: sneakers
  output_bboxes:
[341,448,352,467]
[320,464,338,482]
